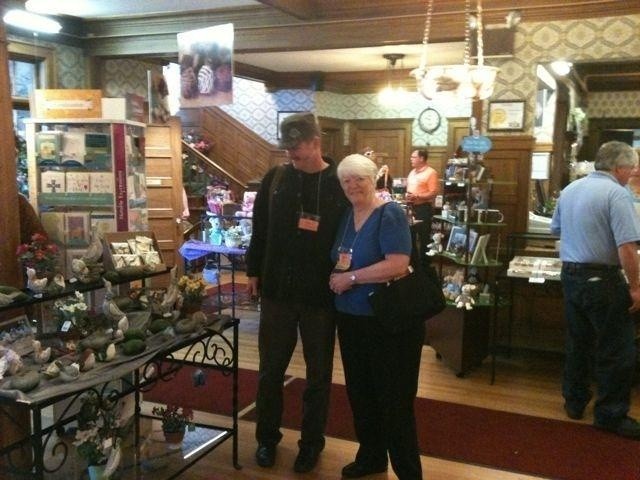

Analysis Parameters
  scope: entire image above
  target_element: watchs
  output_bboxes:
[350,271,357,286]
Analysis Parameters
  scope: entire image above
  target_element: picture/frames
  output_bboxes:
[276,111,309,140]
[488,100,526,132]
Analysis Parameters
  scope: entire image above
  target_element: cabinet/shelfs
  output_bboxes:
[0,266,241,480]
[23,119,147,249]
[424,154,511,378]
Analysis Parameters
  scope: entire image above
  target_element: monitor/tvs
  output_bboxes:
[530,152,552,180]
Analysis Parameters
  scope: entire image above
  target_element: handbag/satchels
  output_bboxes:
[369,267,446,336]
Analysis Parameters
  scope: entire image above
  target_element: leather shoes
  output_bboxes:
[294,450,317,471]
[256,444,275,467]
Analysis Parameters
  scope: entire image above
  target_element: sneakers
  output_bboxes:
[594,415,640,439]
[342,463,388,477]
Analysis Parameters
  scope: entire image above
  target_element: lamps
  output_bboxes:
[378,54,408,107]
[409,1,499,101]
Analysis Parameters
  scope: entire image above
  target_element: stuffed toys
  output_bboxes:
[425,232,444,257]
[235,190,258,218]
[209,217,225,237]
[444,268,463,300]
[455,285,476,310]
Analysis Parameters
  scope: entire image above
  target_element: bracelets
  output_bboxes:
[416,194,420,200]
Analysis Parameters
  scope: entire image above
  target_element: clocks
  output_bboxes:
[417,107,441,134]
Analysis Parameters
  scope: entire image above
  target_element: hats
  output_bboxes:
[279,114,317,149]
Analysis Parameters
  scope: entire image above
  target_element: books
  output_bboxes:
[508,268,560,283]
[506,255,562,269]
[34,130,149,333]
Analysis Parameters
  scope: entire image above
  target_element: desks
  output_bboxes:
[491,231,640,382]
[178,238,247,318]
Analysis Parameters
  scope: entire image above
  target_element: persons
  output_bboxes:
[244,113,352,473]
[551,137,640,439]
[330,153,426,480]
[404,148,440,250]
[15,135,52,243]
